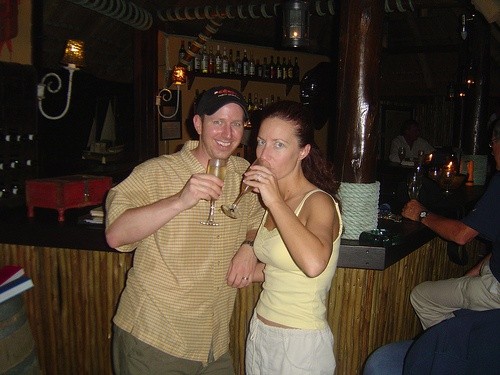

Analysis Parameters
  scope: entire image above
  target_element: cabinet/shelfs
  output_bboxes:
[143,30,337,170]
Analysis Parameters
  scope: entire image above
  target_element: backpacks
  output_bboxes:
[402,307,500,375]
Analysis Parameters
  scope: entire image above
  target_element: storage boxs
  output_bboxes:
[23,174,112,222]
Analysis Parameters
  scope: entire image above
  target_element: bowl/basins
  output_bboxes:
[428,172,470,202]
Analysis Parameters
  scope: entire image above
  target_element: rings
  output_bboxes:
[242,277,248,280]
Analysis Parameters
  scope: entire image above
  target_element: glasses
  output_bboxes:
[488,140,500,150]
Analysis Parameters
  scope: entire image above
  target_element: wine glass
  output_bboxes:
[399,147,406,164]
[221,158,271,219]
[200,158,226,226]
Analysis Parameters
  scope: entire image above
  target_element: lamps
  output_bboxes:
[281,0,311,48]
[154,65,186,119]
[35,38,86,120]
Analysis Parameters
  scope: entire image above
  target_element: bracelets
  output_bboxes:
[242,240,255,248]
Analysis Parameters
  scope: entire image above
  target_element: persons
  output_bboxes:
[105,87,264,375]
[243,102,344,375]
[362,119,500,375]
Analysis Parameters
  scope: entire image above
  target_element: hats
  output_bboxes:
[197,86,250,123]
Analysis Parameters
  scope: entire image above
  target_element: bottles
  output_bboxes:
[179,41,301,127]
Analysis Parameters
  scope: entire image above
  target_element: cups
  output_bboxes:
[405,182,422,203]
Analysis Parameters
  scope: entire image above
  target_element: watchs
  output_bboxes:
[418,209,430,223]
